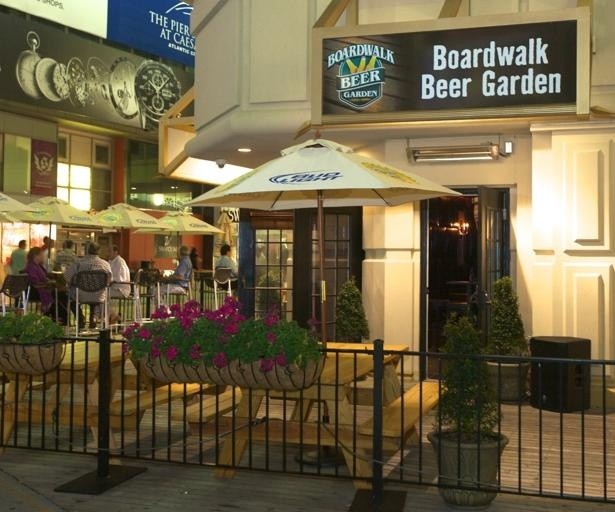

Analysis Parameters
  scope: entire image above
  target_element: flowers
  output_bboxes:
[122,296,316,372]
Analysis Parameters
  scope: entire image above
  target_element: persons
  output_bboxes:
[254,237,269,276]
[217,244,243,301]
[189,248,203,271]
[8,235,161,338]
[156,245,194,316]
[276,238,289,280]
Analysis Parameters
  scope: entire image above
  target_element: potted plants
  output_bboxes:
[0,312,65,374]
[426,313,509,508]
[487,278,531,402]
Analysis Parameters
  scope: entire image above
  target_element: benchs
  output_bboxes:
[85,383,217,456]
[340,380,455,491]
[0,379,48,448]
[166,386,265,480]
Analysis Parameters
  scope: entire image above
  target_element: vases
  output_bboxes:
[129,349,325,390]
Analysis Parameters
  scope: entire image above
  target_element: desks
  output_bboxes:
[4,340,129,451]
[213,340,411,491]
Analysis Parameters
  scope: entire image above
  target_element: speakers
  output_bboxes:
[530,336,591,413]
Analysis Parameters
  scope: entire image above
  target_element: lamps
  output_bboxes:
[404,133,502,164]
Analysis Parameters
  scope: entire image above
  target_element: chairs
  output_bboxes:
[0,265,238,336]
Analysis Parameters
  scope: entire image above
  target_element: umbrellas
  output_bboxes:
[0,190,225,267]
[183,129,463,467]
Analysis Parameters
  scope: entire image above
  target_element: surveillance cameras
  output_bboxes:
[216,158,226,168]
